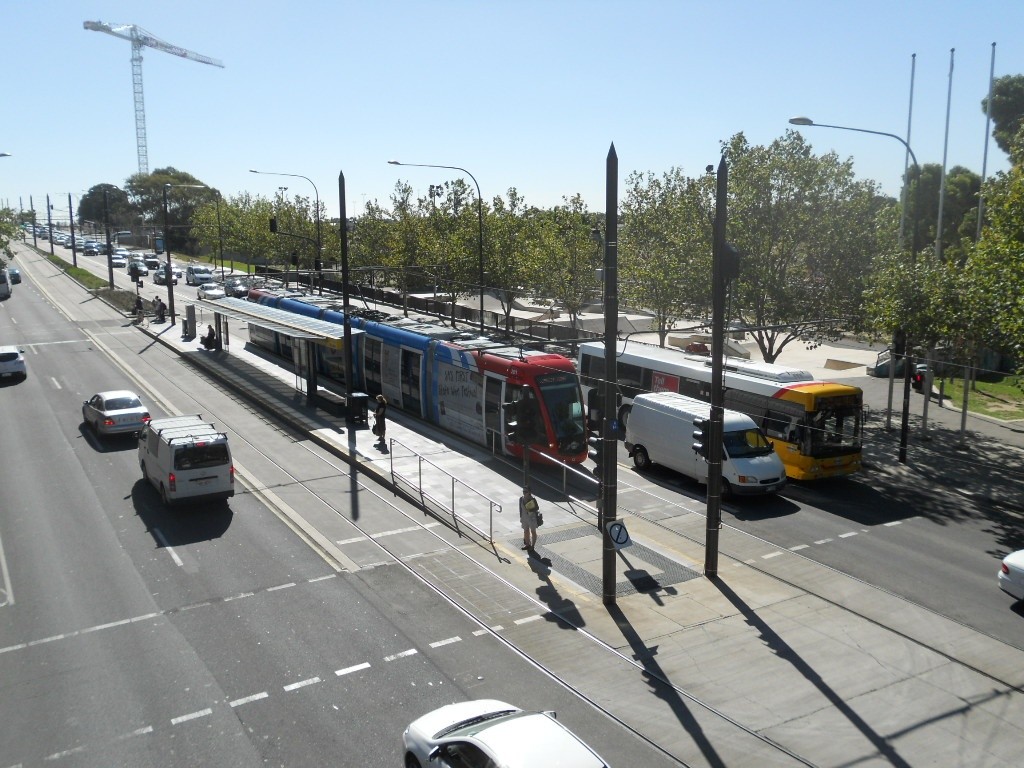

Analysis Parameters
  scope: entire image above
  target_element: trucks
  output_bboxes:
[624,392,787,500]
[111,231,131,242]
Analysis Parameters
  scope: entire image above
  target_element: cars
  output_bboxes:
[402,699,611,768]
[18,222,113,256]
[111,248,182,285]
[8,269,21,284]
[225,278,303,303]
[197,283,225,300]
[82,390,151,439]
[997,549,1024,601]
[0,345,26,380]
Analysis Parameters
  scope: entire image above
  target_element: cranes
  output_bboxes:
[84,21,225,221]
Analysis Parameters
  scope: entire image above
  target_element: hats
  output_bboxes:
[208,325,212,328]
[522,485,531,491]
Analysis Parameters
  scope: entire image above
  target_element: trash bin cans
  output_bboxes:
[345,393,370,425]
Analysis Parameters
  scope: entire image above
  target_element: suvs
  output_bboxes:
[132,414,235,508]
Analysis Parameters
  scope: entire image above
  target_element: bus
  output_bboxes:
[579,339,870,482]
[247,287,588,466]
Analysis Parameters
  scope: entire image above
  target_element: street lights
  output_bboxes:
[249,170,322,296]
[279,186,288,207]
[388,161,484,336]
[789,117,921,462]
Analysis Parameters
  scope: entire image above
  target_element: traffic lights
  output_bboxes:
[588,436,603,478]
[692,418,708,459]
[912,370,924,390]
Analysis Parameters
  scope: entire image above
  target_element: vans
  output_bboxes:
[186,266,213,285]
[0,269,12,299]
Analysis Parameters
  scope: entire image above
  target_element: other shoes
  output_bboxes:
[528,546,534,552]
[521,545,531,550]
[378,437,384,441]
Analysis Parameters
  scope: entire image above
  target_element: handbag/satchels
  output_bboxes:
[372,424,377,435]
[536,514,544,527]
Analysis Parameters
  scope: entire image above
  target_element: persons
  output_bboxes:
[371,395,387,442]
[204,324,214,348]
[152,295,165,323]
[519,485,539,552]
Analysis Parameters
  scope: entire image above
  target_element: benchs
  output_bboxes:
[318,390,346,413]
